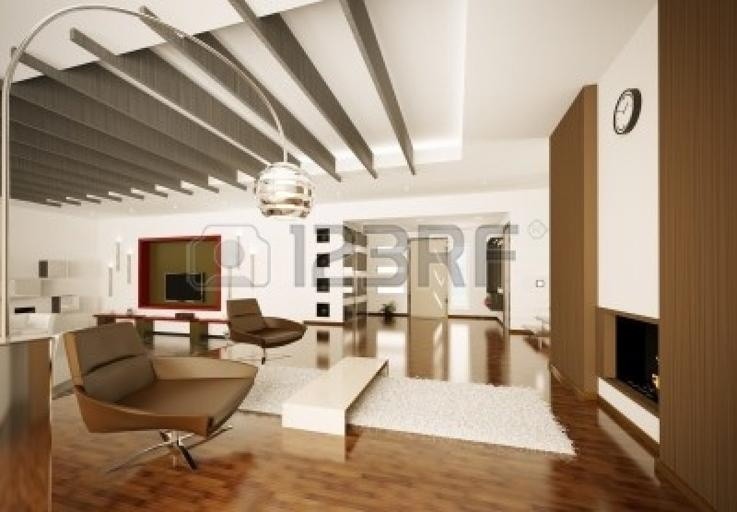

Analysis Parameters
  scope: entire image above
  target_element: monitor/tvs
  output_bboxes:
[164,271,204,304]
[613,314,659,404]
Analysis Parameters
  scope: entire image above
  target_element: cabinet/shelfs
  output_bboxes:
[11,259,80,313]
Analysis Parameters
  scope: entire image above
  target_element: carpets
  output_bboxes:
[237,360,577,456]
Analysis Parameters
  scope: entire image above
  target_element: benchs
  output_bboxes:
[521,323,550,350]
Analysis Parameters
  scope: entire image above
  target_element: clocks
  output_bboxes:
[613,88,641,134]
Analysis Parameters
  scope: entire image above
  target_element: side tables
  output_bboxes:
[534,316,549,346]
[281,356,389,436]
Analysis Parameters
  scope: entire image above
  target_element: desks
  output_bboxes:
[93,314,146,330]
[142,317,229,350]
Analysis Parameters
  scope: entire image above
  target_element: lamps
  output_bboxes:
[2,4,313,348]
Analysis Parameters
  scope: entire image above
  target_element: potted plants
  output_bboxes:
[379,304,396,322]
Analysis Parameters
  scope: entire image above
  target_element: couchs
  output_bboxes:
[9,312,97,399]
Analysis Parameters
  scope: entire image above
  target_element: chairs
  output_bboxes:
[64,322,258,474]
[227,298,307,365]
